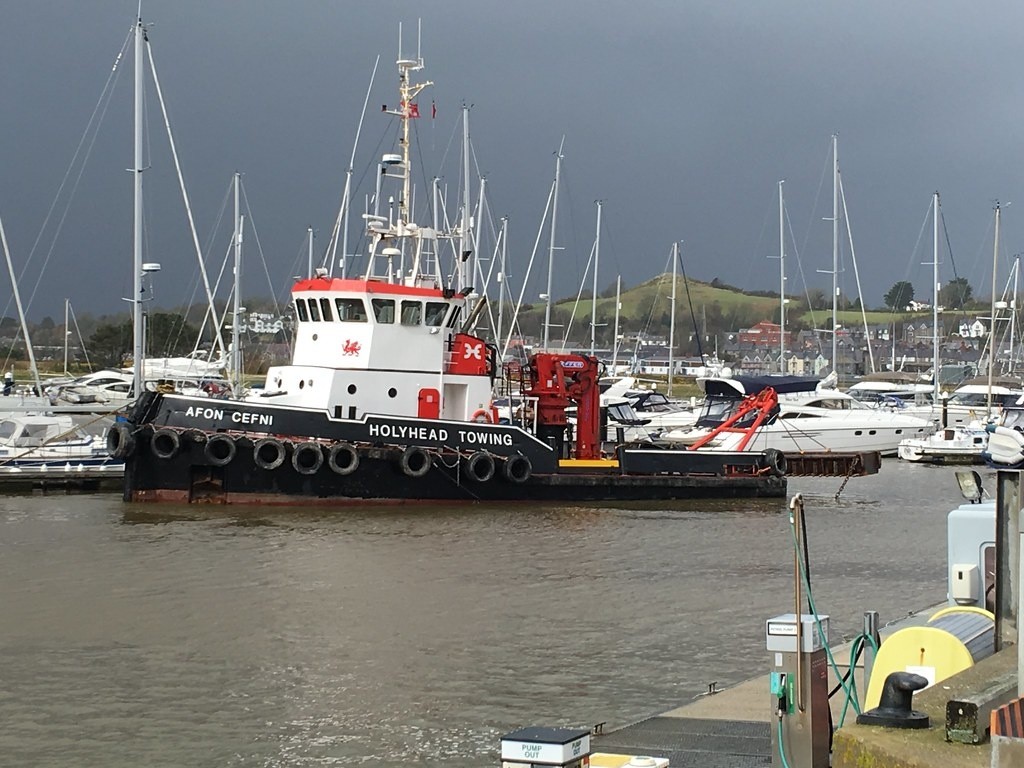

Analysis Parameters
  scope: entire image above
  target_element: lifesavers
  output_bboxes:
[151,428,181,463]
[254,437,285,471]
[764,449,788,476]
[329,442,359,476]
[292,441,324,475]
[470,409,492,424]
[503,454,533,485]
[402,445,432,477]
[466,452,496,483]
[204,434,236,468]
[107,421,136,463]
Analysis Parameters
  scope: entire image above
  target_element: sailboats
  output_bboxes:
[434,103,1024,468]
[0,0,314,466]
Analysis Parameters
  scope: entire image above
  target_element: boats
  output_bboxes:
[106,18,788,508]
